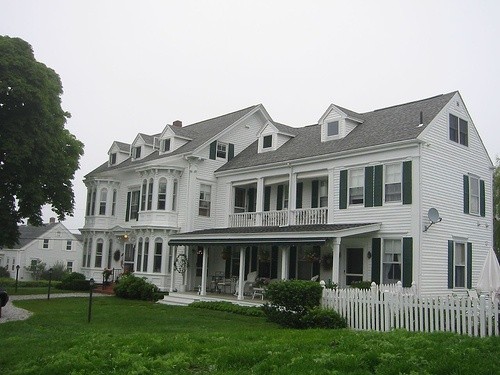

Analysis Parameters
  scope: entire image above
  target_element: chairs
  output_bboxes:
[234,271,258,298]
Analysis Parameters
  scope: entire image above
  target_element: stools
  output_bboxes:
[252,287,265,300]
[218,282,232,295]
